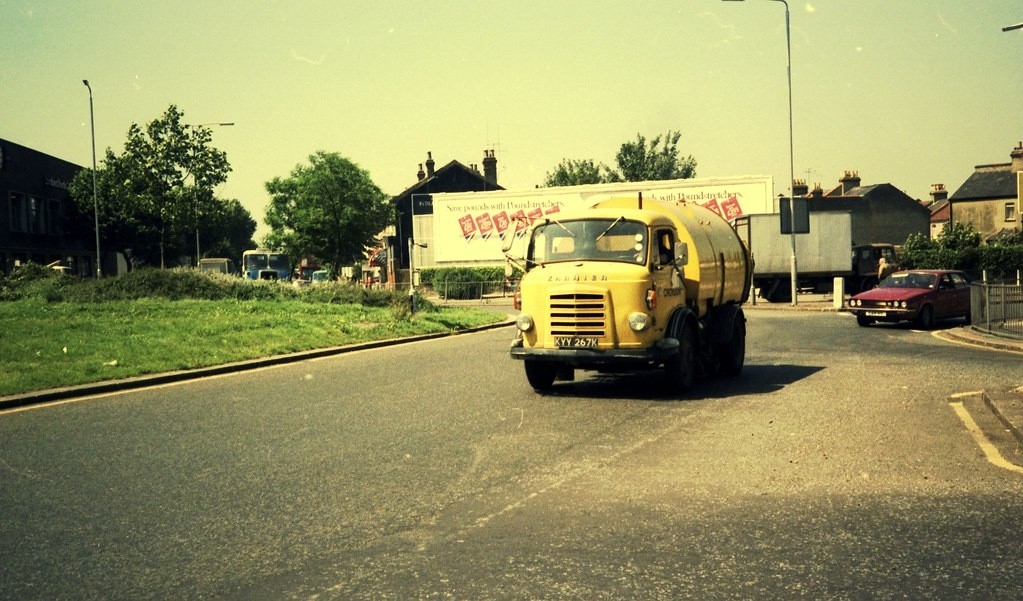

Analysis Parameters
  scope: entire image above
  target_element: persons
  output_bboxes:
[878,258,892,284]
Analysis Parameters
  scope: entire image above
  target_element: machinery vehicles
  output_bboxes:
[500,190,756,394]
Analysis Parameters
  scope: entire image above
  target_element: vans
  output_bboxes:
[197,258,235,276]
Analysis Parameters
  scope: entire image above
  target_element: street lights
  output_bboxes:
[82,80,102,280]
[190,122,235,269]
[720,0,797,306]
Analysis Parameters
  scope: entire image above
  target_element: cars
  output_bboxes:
[837,270,988,331]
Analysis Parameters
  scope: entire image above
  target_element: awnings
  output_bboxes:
[369,249,386,266]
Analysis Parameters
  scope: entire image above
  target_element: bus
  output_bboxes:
[242,249,292,284]
